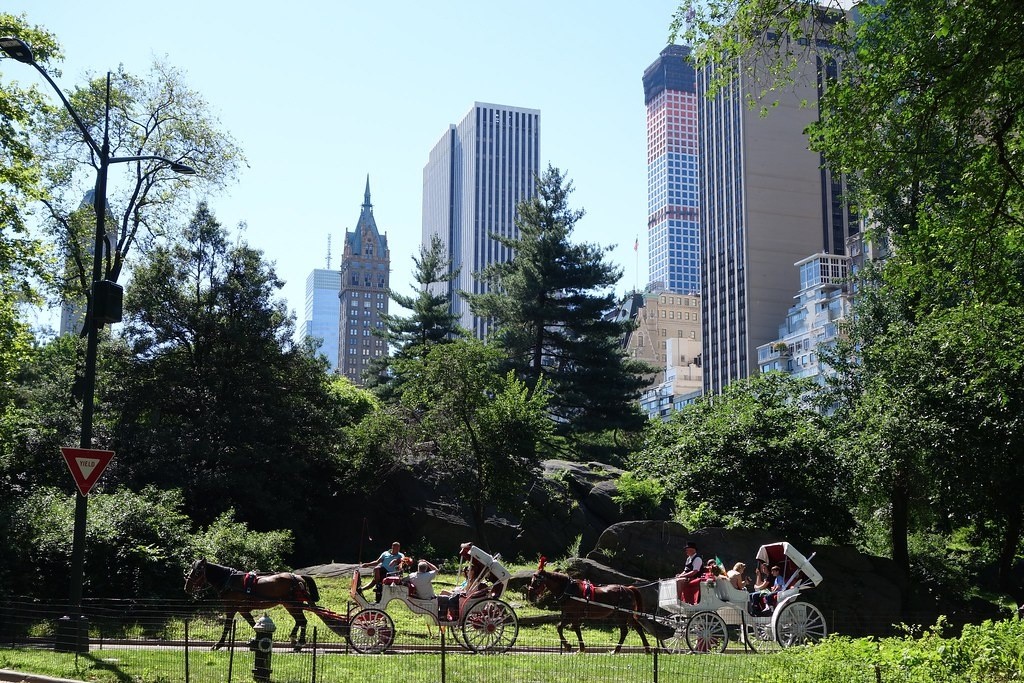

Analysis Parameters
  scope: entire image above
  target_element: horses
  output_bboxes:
[528,569,651,655]
[184,556,320,653]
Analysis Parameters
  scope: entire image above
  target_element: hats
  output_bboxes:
[707,559,716,566]
[683,542,696,549]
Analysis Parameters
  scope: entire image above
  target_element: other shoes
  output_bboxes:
[452,616,459,621]
[373,588,382,593]
[761,604,774,613]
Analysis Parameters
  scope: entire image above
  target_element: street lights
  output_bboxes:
[0,36,198,654]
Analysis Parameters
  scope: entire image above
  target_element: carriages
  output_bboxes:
[184,540,520,656]
[526,541,828,656]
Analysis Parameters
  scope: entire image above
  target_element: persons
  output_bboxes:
[707,558,783,617]
[676,541,705,603]
[409,559,450,622]
[440,542,504,622]
[357,541,405,594]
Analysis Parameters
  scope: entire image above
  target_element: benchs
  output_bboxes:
[383,574,401,585]
[716,574,750,613]
[458,583,490,618]
[410,590,439,620]
[757,584,798,615]
[689,575,714,606]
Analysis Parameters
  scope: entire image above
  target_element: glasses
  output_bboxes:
[467,570,473,571]
